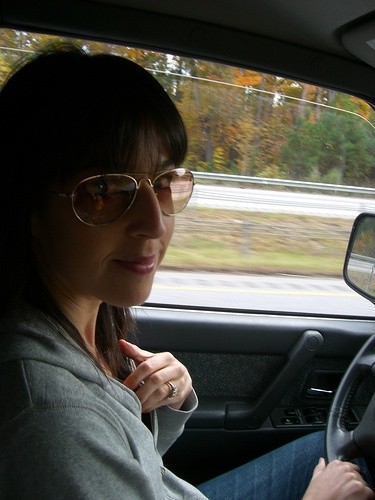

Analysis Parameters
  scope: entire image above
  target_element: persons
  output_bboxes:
[0,41,374,500]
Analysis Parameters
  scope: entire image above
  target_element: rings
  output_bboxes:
[167,382,177,399]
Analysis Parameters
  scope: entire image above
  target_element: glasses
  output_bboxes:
[48,168,195,227]
